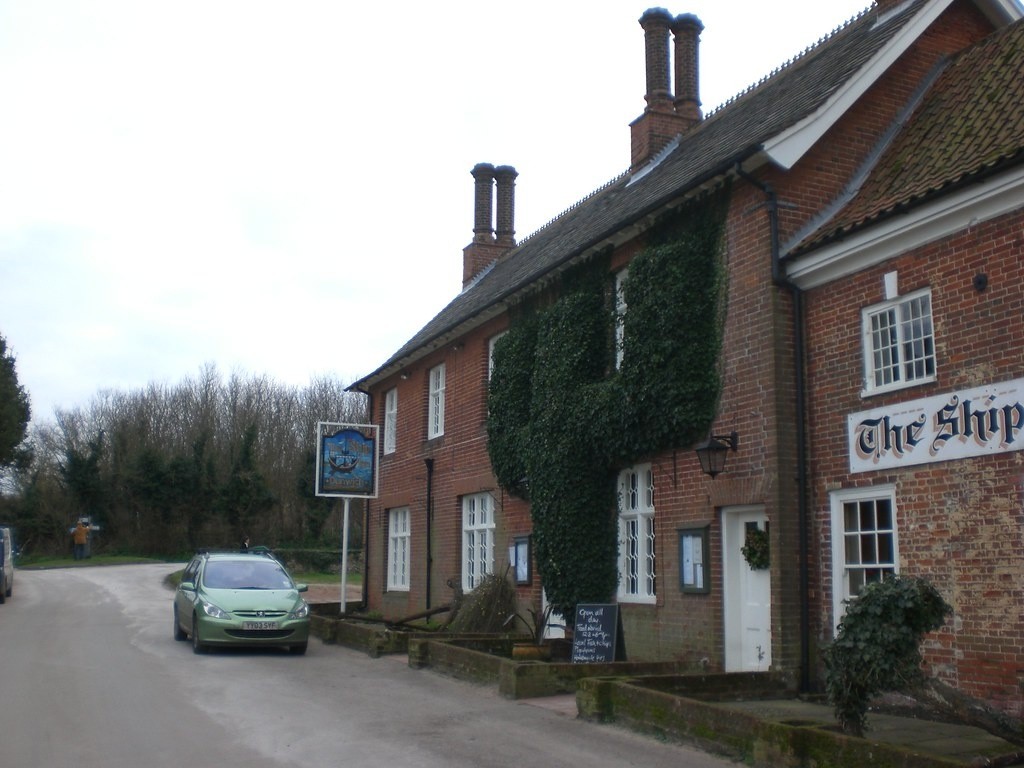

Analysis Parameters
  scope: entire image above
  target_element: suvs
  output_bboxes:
[172,546,311,654]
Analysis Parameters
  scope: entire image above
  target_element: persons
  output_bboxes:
[240,536,249,554]
[71,522,93,560]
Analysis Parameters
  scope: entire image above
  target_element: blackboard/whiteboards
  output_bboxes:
[571,602,619,664]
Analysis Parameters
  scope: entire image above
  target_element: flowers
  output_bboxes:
[504,603,560,645]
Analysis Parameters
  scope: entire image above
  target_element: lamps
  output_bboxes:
[518,476,530,499]
[693,432,738,480]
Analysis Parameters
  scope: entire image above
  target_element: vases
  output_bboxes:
[513,645,553,659]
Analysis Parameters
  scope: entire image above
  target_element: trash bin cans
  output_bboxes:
[246,546,269,556]
